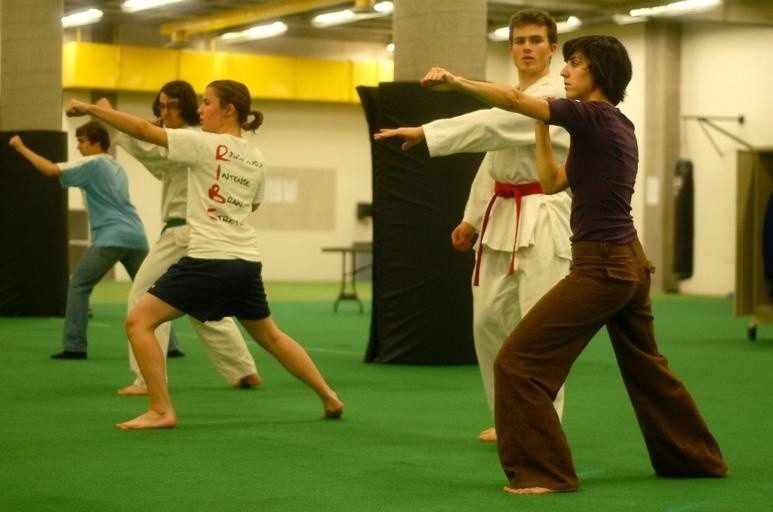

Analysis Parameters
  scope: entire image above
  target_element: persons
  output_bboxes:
[369,8,577,443]
[92,77,262,398]
[64,77,346,430]
[7,118,186,360]
[418,35,729,496]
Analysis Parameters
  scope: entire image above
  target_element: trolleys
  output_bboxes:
[734,144,772,342]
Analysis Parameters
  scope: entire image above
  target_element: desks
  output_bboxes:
[322,242,373,316]
